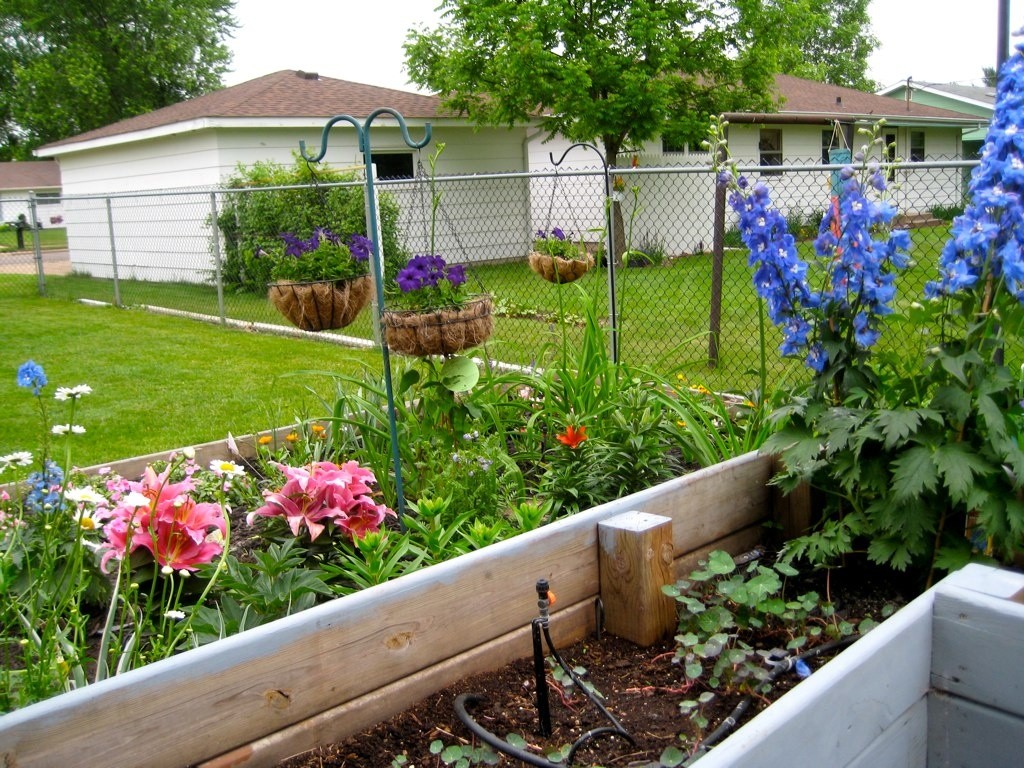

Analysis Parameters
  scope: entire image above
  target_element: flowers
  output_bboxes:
[0,24,1024,717]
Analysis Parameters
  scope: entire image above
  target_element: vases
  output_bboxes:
[528,251,594,284]
[0,370,780,768]
[267,274,376,333]
[380,294,495,356]
